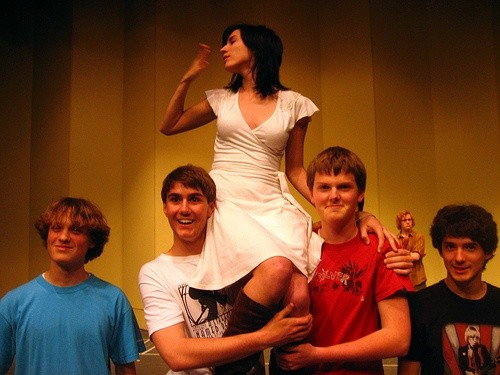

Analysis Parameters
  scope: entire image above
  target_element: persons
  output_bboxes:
[270,146,413,375]
[0,198,145,375]
[160,24,400,375]
[399,205,500,375]
[391,209,429,293]
[137,165,414,375]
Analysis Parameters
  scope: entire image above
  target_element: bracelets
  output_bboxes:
[356,214,375,224]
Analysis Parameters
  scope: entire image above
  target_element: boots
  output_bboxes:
[269,340,308,375]
[211,284,277,375]
